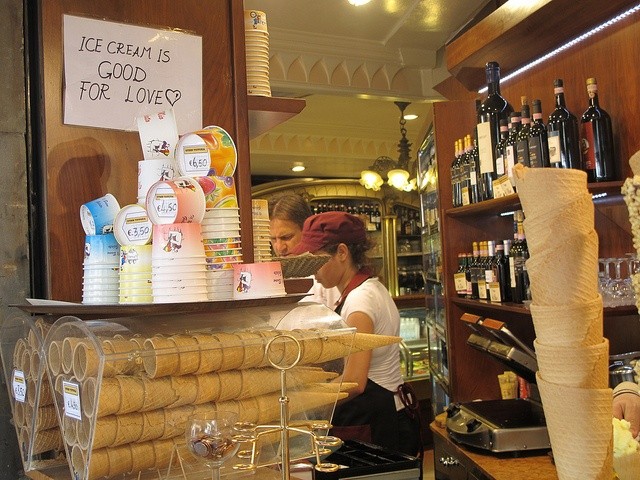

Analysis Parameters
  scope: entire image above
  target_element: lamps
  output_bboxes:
[359,101,418,192]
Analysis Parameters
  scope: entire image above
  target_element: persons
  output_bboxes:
[290,212,422,456]
[268,194,342,311]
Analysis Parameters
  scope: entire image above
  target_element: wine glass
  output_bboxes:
[184,410,240,480]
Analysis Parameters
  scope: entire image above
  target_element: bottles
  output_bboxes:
[452,54,618,306]
[309,196,381,232]
[394,207,421,235]
[398,264,423,295]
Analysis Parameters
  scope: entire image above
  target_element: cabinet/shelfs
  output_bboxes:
[367,12,640,480]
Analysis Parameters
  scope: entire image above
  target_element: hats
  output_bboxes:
[291,211,368,255]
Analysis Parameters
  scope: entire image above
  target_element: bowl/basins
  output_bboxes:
[78,122,287,305]
[244,8,271,96]
